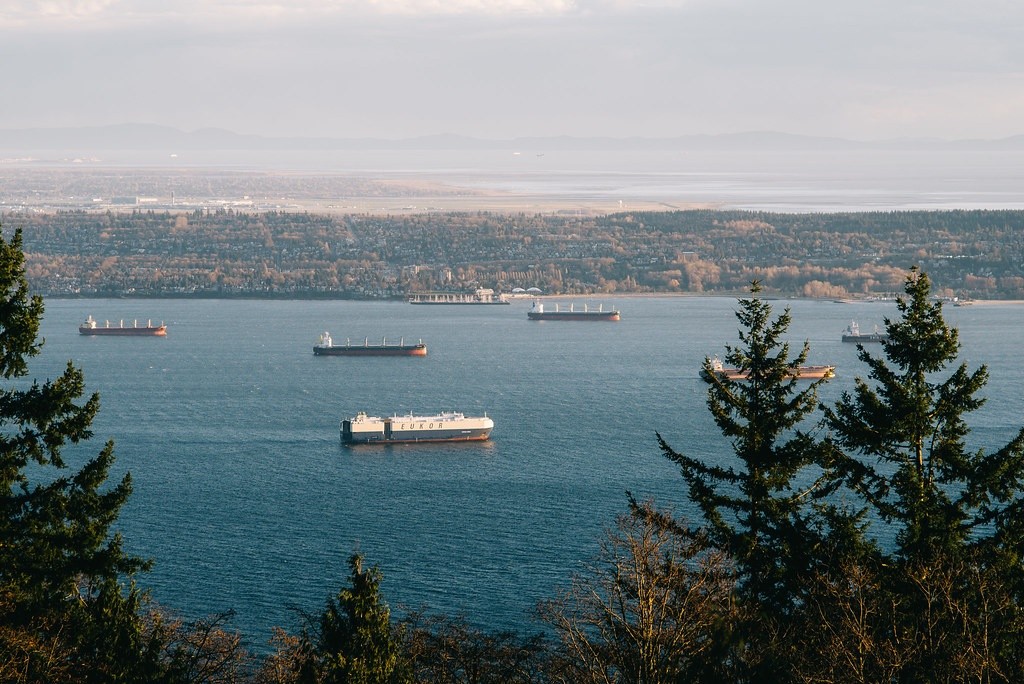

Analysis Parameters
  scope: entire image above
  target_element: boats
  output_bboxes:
[313,331,426,356]
[78,315,167,337]
[527,298,620,321]
[409,288,511,305]
[953,299,972,306]
[841,320,891,342]
[700,353,835,383]
[339,412,494,444]
